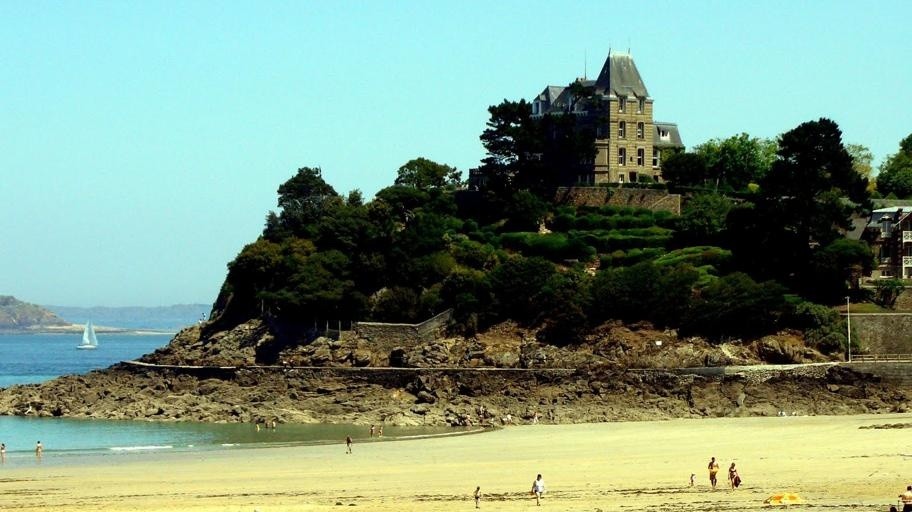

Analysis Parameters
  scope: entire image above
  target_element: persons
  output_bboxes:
[198,312,206,324]
[902,485,912,512]
[455,407,512,426]
[532,474,543,507]
[379,426,383,436]
[474,488,483,508]
[728,463,738,489]
[533,411,539,424]
[1,444,6,462]
[708,457,718,488]
[369,425,375,437]
[345,434,352,453]
[254,420,276,431]
[36,440,42,456]
[690,473,696,485]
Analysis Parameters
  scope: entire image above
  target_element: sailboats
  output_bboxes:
[75,319,99,349]
